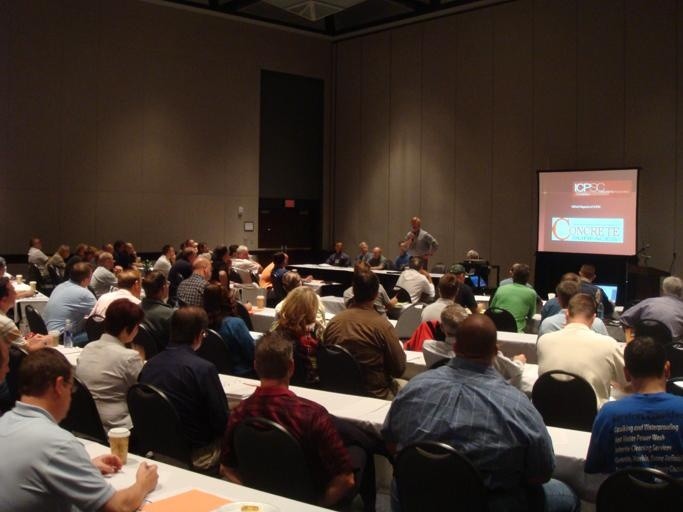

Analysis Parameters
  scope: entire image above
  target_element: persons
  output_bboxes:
[65,239,138,254]
[90,252,118,297]
[537,293,629,411]
[27,238,49,275]
[326,241,351,267]
[137,306,229,440]
[354,241,371,265]
[323,270,406,402]
[539,282,609,337]
[212,240,263,283]
[0,276,19,343]
[540,272,580,316]
[488,265,536,333]
[0,338,10,384]
[75,299,144,453]
[267,286,325,389]
[367,246,389,270]
[423,303,521,389]
[0,347,159,512]
[203,284,258,380]
[421,273,460,322]
[584,337,683,512]
[259,252,287,284]
[450,263,485,313]
[177,238,212,256]
[45,245,70,279]
[41,263,97,345]
[137,271,175,358]
[0,257,34,297]
[620,276,682,356]
[220,332,355,508]
[177,257,211,307]
[499,263,532,289]
[580,265,614,316]
[274,271,325,323]
[393,257,436,303]
[380,314,557,512]
[152,244,175,280]
[400,217,439,271]
[86,271,141,348]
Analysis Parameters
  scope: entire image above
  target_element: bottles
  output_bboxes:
[63,318,74,347]
[596,301,605,320]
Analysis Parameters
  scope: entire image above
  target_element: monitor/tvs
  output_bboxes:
[468,274,487,288]
[594,284,618,306]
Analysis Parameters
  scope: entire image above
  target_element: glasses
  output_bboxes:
[60,380,79,395]
[202,330,209,340]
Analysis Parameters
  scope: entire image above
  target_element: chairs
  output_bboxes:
[32,263,56,290]
[483,307,518,333]
[85,314,105,342]
[224,417,302,494]
[595,466,683,512]
[315,344,359,395]
[47,264,56,288]
[390,441,488,511]
[198,329,233,371]
[25,304,48,335]
[125,382,182,457]
[134,323,151,350]
[388,284,411,303]
[531,369,598,432]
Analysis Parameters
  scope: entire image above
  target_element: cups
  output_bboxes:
[256,296,264,309]
[16,274,23,285]
[107,428,131,465]
[30,281,37,290]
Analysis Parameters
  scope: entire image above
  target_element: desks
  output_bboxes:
[288,262,469,299]
[7,274,50,328]
[320,295,428,319]
[20,335,610,507]
[75,435,335,512]
[532,313,626,341]
[496,329,626,368]
[248,303,276,332]
[402,350,539,396]
